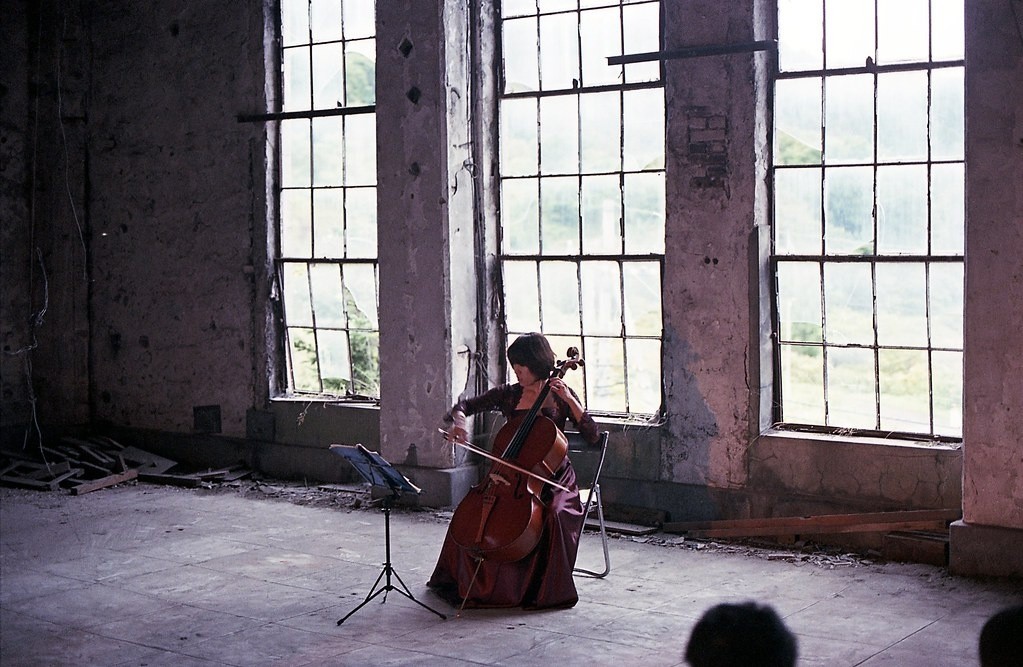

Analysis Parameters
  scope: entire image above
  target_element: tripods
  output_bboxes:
[327,444,448,625]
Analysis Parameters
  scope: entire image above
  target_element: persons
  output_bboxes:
[681,598,801,667]
[978,602,1023,667]
[423,329,603,611]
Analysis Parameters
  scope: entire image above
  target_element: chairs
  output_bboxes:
[563,432,610,578]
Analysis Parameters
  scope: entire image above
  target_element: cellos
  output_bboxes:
[447,347,585,618]
